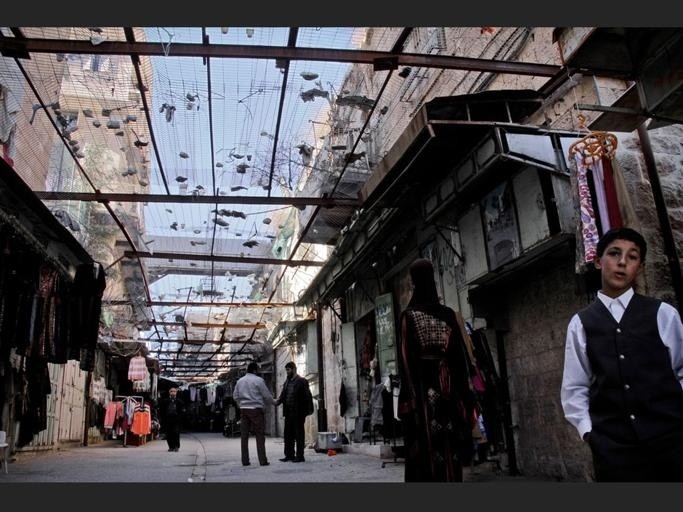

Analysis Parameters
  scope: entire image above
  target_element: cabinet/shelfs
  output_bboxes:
[317,430,343,453]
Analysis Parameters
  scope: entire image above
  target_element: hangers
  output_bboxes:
[92,370,101,383]
[131,349,142,357]
[131,400,149,413]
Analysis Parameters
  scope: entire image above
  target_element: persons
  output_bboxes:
[274,362,313,462]
[559,224,683,483]
[232,362,279,465]
[156,386,187,452]
[396,256,480,483]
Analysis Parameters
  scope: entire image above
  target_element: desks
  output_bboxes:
[350,415,371,442]
